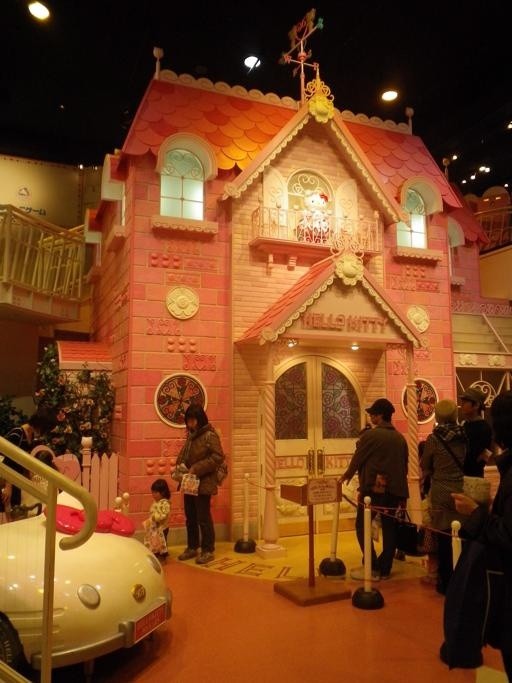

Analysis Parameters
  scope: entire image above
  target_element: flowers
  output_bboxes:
[30,344,117,457]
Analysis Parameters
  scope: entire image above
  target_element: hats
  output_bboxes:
[435,398,459,423]
[365,398,396,415]
[457,388,481,403]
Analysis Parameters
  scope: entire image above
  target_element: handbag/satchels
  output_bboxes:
[180,474,200,496]
[143,518,168,554]
[395,507,419,554]
[417,512,434,554]
[216,463,228,484]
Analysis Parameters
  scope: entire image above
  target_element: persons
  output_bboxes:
[336,398,409,580]
[147,478,171,559]
[459,387,492,478]
[287,8,317,76]
[449,391,511,683]
[420,399,466,595]
[0,406,58,512]
[176,405,225,565]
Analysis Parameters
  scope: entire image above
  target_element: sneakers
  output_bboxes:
[420,575,438,586]
[380,572,391,580]
[350,566,381,582]
[195,551,215,564]
[178,548,198,561]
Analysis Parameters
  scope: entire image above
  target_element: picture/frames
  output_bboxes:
[402,378,439,424]
[152,372,209,430]
